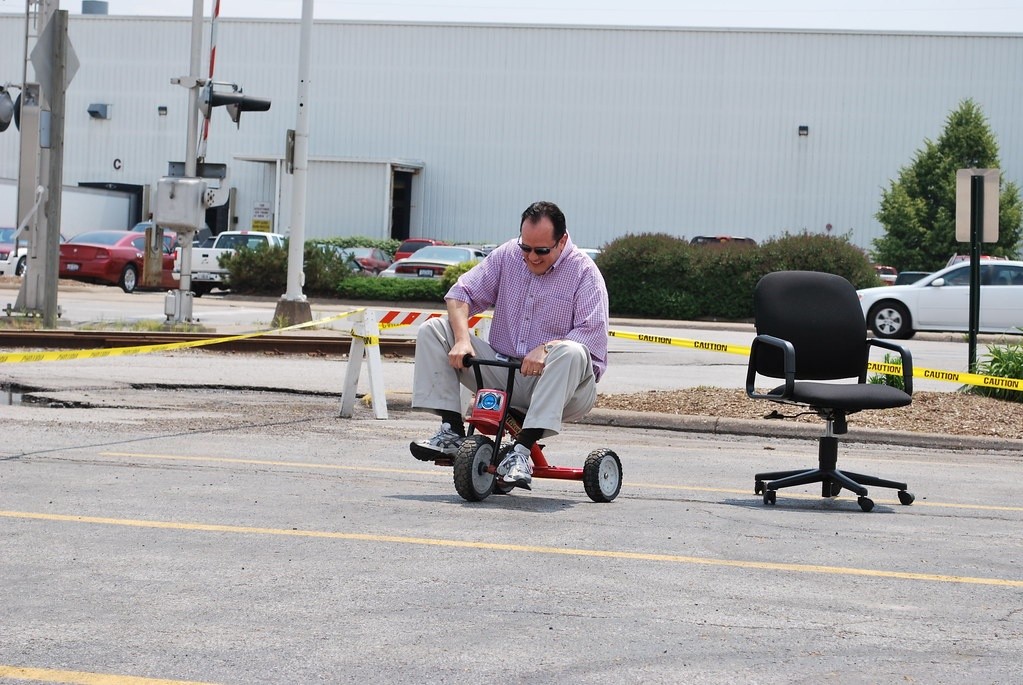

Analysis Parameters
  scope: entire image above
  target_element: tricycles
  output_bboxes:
[434,352,624,503]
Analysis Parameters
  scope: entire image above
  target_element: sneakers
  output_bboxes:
[496,444,533,482]
[410,422,466,462]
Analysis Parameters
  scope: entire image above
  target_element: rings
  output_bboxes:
[532,371,539,374]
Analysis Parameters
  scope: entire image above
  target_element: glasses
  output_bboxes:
[517,233,562,255]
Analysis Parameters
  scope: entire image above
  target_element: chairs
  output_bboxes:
[745,270,916,511]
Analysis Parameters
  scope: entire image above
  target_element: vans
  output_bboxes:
[394,237,445,264]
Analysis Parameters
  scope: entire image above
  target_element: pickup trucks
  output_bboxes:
[171,230,288,295]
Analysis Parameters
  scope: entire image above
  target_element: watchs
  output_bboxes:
[542,342,553,352]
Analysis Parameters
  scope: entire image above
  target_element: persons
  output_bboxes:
[410,201,611,484]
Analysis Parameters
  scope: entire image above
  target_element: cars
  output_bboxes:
[59,230,180,293]
[343,246,392,276]
[131,220,364,273]
[873,265,897,286]
[0,223,66,279]
[377,245,489,279]
[857,259,1023,340]
